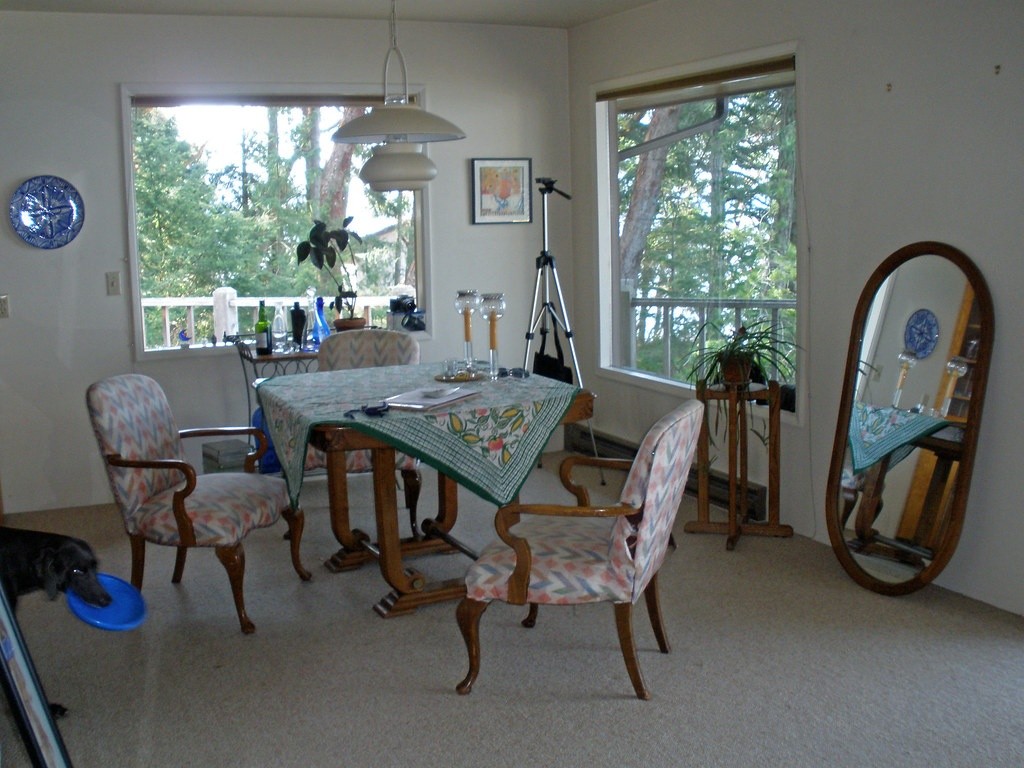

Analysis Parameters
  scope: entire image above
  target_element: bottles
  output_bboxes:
[273,301,287,353]
[290,301,304,345]
[312,296,330,347]
[300,287,323,351]
[255,299,273,355]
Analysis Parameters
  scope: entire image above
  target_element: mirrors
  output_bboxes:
[824,240,995,599]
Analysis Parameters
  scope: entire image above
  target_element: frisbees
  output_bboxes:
[64,572,149,632]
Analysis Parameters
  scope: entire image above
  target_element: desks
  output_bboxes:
[681,377,793,551]
[846,402,967,558]
[242,347,317,452]
[253,359,596,620]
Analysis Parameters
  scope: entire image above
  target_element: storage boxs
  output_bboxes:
[202,439,254,476]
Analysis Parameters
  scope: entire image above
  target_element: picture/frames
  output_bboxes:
[471,158,533,224]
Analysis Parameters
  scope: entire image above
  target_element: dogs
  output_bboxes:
[0,526,112,721]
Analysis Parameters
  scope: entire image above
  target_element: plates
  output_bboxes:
[435,373,481,381]
[66,573,148,630]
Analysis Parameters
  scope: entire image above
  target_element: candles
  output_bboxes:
[463,305,471,342]
[489,308,496,349]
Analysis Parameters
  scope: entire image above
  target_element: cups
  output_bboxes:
[464,358,478,378]
[928,408,938,417]
[441,357,458,378]
[915,403,924,413]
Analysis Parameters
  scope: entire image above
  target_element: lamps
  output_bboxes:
[330,1,467,191]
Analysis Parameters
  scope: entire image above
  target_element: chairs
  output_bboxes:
[455,399,705,702]
[85,374,313,635]
[282,327,424,537]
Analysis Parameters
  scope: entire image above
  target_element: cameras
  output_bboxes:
[390,295,417,313]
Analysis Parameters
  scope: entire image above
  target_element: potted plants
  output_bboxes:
[297,216,367,332]
[672,317,810,471]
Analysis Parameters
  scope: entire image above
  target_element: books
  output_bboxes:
[380,387,479,413]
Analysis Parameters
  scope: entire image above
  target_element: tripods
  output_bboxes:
[522,178,607,487]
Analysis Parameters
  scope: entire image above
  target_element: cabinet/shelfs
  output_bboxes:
[895,281,983,548]
